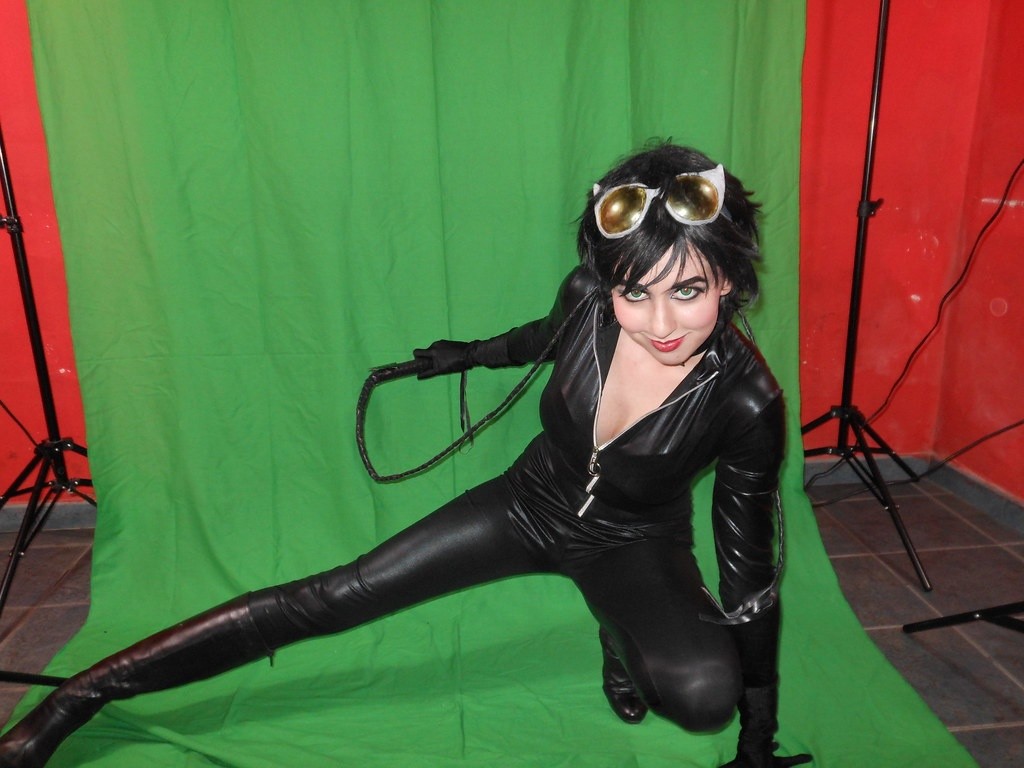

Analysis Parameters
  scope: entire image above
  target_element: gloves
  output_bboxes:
[717,723,812,768]
[412,338,480,380]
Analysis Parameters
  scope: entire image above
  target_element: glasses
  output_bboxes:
[592,163,735,238]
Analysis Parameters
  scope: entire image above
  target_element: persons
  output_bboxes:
[0,136,814,768]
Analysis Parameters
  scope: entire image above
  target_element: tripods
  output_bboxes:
[0,132,100,619]
[802,0,932,590]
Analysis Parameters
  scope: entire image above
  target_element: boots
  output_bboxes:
[0,587,276,768]
[599,623,667,724]
